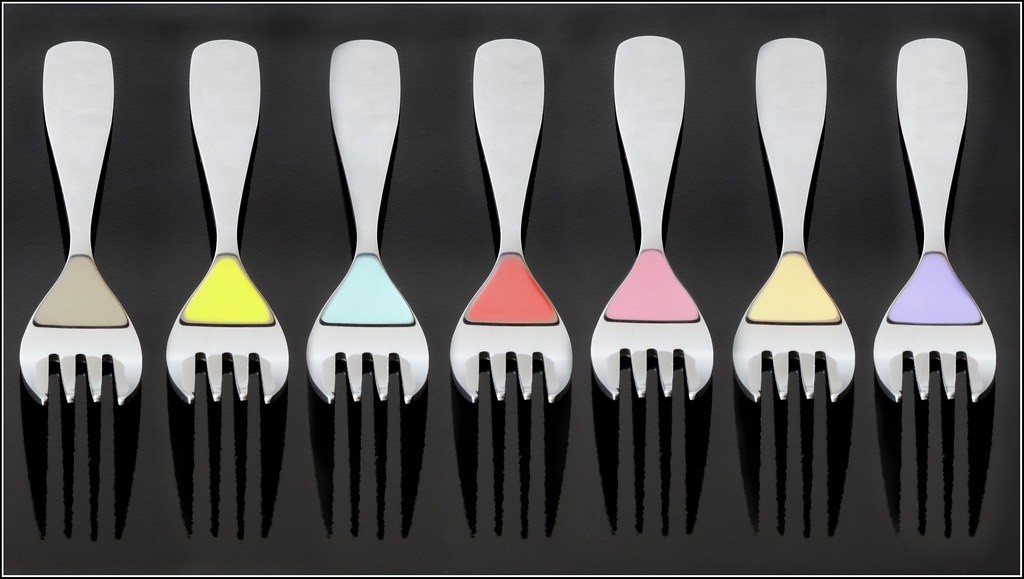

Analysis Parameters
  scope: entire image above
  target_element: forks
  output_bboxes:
[166,40,290,405]
[733,37,856,403]
[591,36,714,400]
[449,39,573,402]
[306,39,430,402]
[873,38,997,403]
[19,41,143,405]
